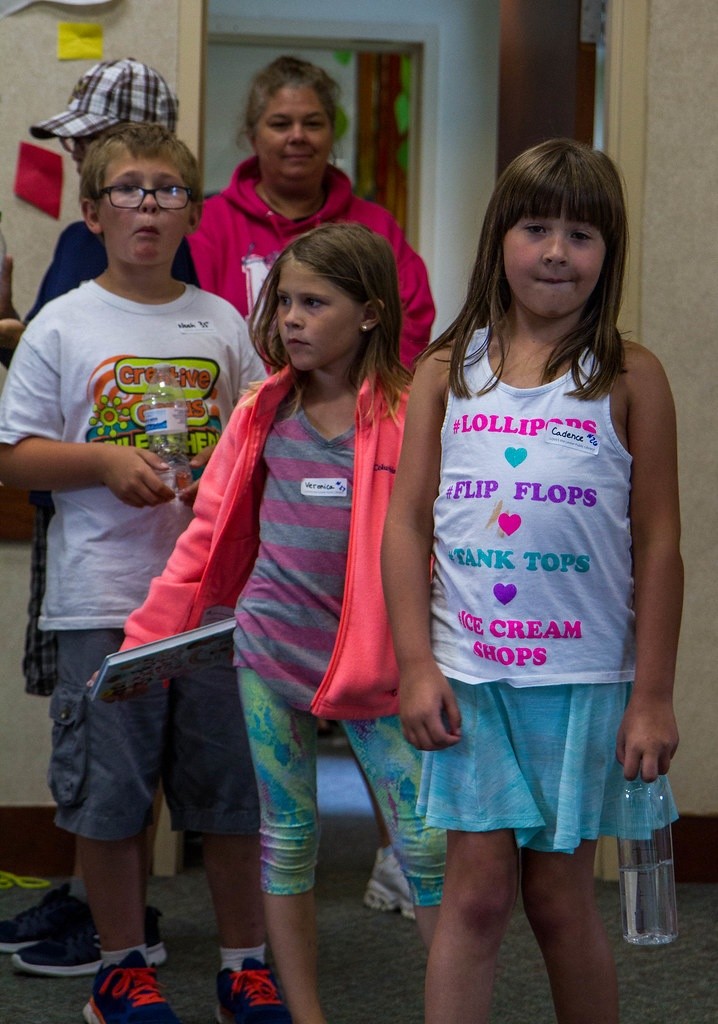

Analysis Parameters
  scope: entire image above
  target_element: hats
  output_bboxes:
[29,57,179,140]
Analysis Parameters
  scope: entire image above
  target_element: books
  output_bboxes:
[87,616,239,704]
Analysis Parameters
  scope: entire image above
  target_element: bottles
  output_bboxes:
[143,364,193,503]
[616,770,679,945]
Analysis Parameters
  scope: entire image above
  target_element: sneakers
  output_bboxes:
[0,881,95,953]
[82,950,182,1024]
[365,844,415,919]
[215,956,293,1024]
[11,904,167,977]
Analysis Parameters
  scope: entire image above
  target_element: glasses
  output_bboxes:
[93,184,195,210]
[59,136,102,156]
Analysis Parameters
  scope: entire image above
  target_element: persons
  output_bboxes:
[1,121,298,1023]
[381,135,688,1023]
[185,55,439,922]
[3,54,201,971]
[79,222,453,1023]
